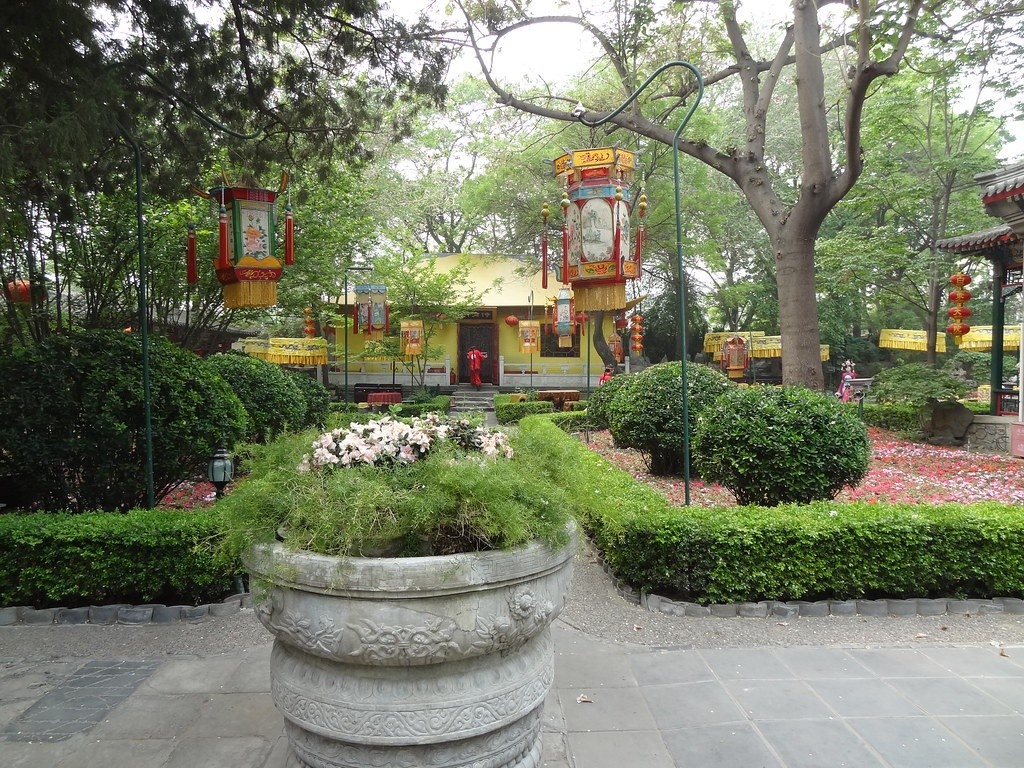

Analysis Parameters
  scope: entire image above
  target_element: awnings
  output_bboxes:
[878,328,945,354]
[703,329,829,364]
[245,338,270,359]
[268,335,328,366]
[956,324,1020,350]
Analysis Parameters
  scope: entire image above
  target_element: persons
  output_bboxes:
[466,344,486,389]
[835,359,857,401]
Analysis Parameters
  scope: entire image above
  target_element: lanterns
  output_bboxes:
[3,279,49,308]
[398,318,423,356]
[945,272,972,345]
[351,281,392,340]
[513,149,650,365]
[304,307,316,336]
[504,314,519,327]
[720,333,749,381]
[187,166,297,310]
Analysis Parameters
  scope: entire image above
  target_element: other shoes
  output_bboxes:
[472,383,481,390]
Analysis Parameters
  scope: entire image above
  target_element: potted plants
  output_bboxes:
[212,410,574,768]
